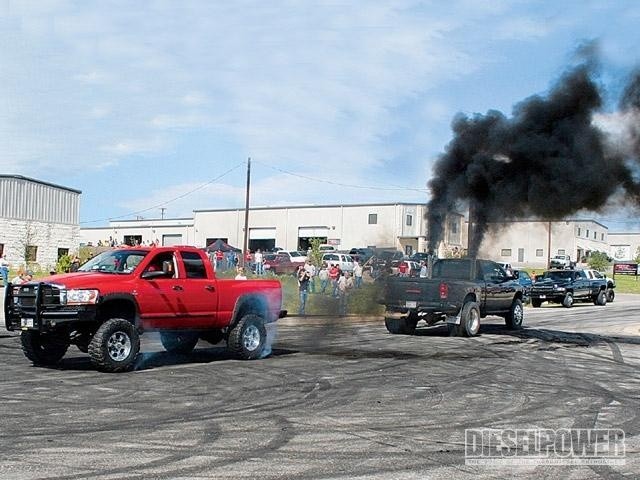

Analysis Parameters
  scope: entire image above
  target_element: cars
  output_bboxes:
[250,243,439,275]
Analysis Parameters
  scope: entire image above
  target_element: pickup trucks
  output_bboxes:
[376,258,533,336]
[530,254,616,307]
[3,245,287,372]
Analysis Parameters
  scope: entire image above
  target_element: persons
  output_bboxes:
[234,266,247,280]
[21,270,34,285]
[87,235,160,249]
[234,248,254,269]
[227,249,235,268]
[72,255,80,269]
[530,270,536,283]
[216,248,223,268]
[297,268,310,316]
[255,249,263,275]
[304,260,317,294]
[319,261,363,315]
[11,273,24,303]
[396,259,428,280]
[261,258,267,265]
[0,254,10,289]
